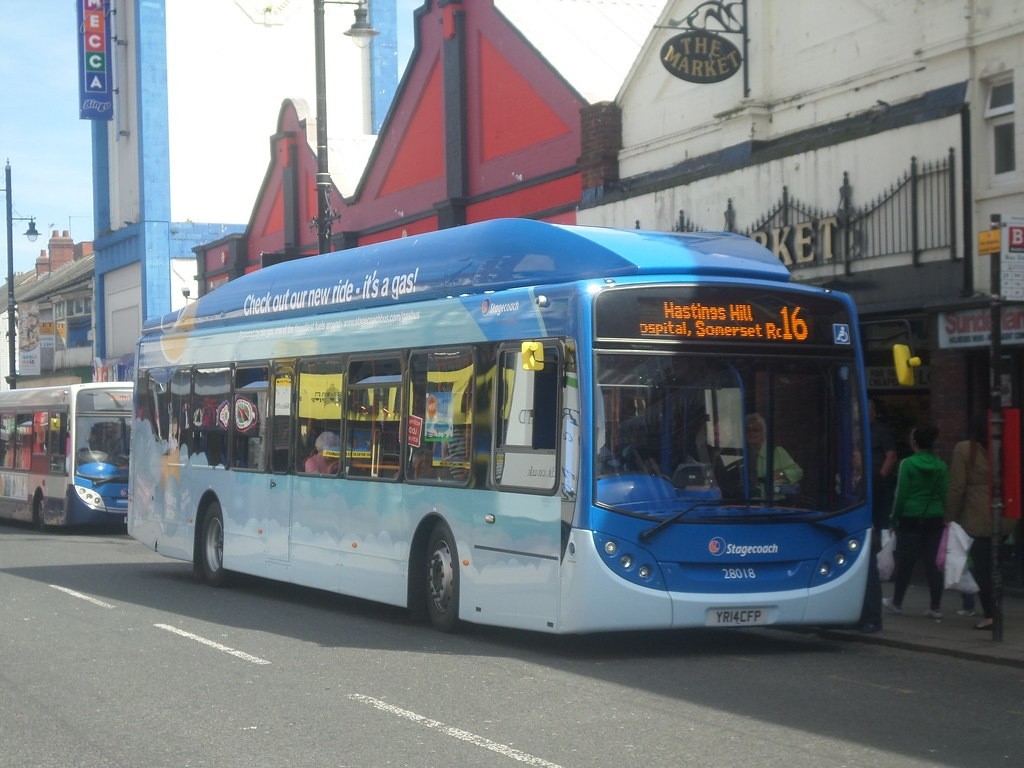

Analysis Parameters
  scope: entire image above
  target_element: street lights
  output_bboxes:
[1,159,41,389]
[312,0,381,256]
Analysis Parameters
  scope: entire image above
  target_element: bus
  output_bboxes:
[0,379,140,529]
[125,216,924,643]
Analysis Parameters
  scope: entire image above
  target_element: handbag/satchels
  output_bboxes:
[936,520,980,595]
[876,529,898,581]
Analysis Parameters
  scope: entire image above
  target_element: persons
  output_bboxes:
[944,406,1016,631]
[305,429,341,474]
[740,412,804,503]
[879,424,953,619]
[834,443,902,558]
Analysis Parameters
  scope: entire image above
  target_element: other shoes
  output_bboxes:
[920,606,942,619]
[977,618,993,630]
[957,609,978,619]
[882,605,899,620]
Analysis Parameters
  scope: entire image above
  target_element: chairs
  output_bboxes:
[302,449,319,472]
[415,457,436,479]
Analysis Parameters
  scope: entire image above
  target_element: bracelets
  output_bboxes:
[776,468,785,482]
